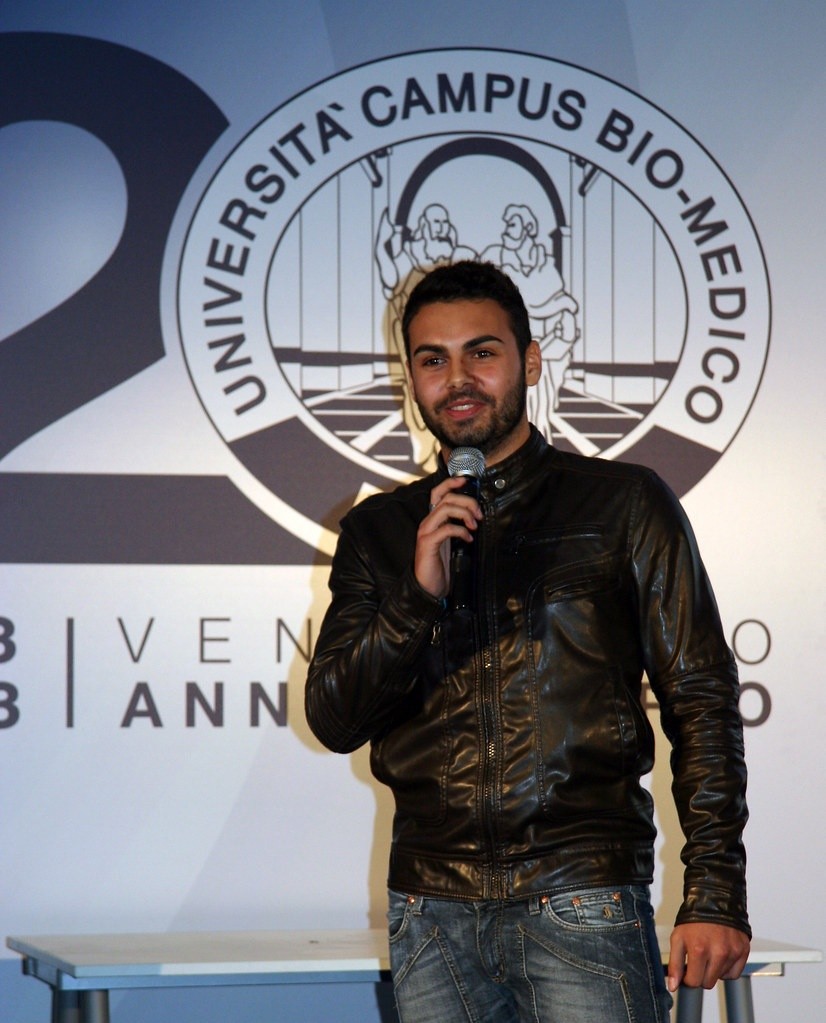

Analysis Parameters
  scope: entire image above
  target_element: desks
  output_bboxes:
[7,933,826,1023]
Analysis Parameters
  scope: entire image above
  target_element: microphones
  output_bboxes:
[445,447,486,581]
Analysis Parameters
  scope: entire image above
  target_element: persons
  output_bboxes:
[302,258,755,1023]
